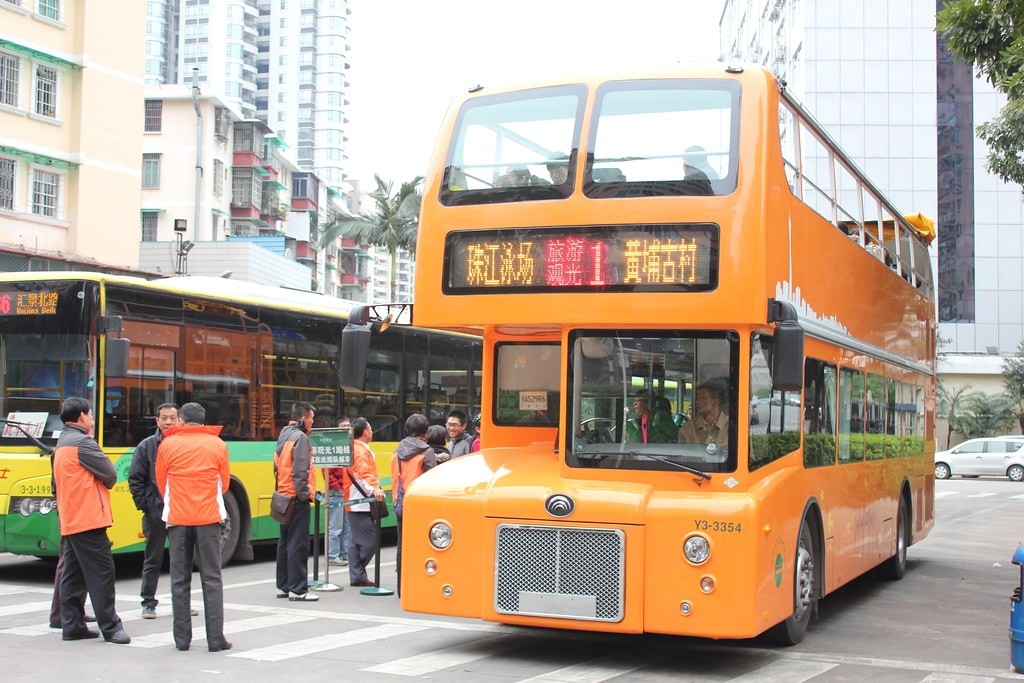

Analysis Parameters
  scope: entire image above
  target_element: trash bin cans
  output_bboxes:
[1009,545,1024,676]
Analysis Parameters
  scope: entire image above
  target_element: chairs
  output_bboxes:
[200,380,479,439]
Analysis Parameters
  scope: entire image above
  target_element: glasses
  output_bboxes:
[445,423,462,428]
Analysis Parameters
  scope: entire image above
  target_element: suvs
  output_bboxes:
[935,435,1024,482]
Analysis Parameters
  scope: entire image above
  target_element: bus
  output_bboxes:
[401,63,939,651]
[0,271,488,564]
[427,371,693,443]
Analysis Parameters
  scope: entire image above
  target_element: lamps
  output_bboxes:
[175,218,187,231]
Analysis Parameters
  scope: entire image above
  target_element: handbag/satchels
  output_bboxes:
[391,454,404,517]
[367,489,389,521]
[269,490,297,527]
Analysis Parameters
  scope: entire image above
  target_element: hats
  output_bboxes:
[471,413,481,423]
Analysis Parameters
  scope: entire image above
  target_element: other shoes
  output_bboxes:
[176,644,188,651]
[208,641,232,652]
[351,580,375,586]
[49,622,62,627]
[82,615,97,622]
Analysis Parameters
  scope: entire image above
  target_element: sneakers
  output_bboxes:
[288,591,319,601]
[141,606,156,618]
[277,588,289,598]
[189,608,198,616]
[329,557,350,566]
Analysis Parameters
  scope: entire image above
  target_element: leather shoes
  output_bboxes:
[62,630,99,639]
[107,629,131,644]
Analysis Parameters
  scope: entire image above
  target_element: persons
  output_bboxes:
[49,397,131,644]
[128,403,199,620]
[155,402,233,652]
[496,175,518,187]
[547,151,569,184]
[470,414,481,452]
[674,405,691,429]
[54,331,122,413]
[273,401,319,602]
[682,146,719,180]
[626,388,680,444]
[849,227,922,288]
[678,383,729,449]
[426,425,451,464]
[838,222,848,235]
[507,163,531,185]
[445,410,472,460]
[391,414,438,600]
[322,415,352,566]
[342,417,386,586]
